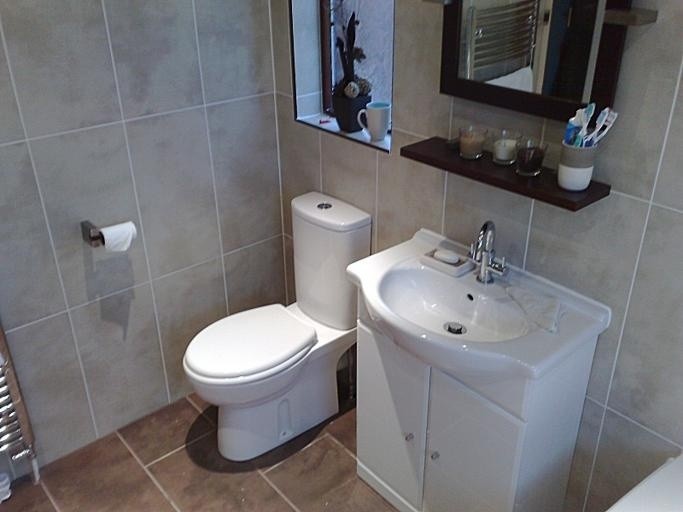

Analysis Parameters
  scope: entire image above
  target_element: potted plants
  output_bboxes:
[324,11,372,134]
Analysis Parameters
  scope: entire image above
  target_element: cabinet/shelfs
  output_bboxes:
[356,319,596,507]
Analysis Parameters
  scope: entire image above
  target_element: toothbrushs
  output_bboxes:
[576,105,619,148]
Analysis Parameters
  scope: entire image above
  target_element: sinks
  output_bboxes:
[378,264,530,344]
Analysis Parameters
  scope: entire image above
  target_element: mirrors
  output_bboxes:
[440,0,609,114]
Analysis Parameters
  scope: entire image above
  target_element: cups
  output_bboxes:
[457,126,490,160]
[513,138,549,177]
[489,127,523,165]
[357,101,391,143]
[558,136,598,193]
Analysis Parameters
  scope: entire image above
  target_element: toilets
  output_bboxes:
[179,192,371,462]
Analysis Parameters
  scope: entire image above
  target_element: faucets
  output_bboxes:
[474,221,495,281]
